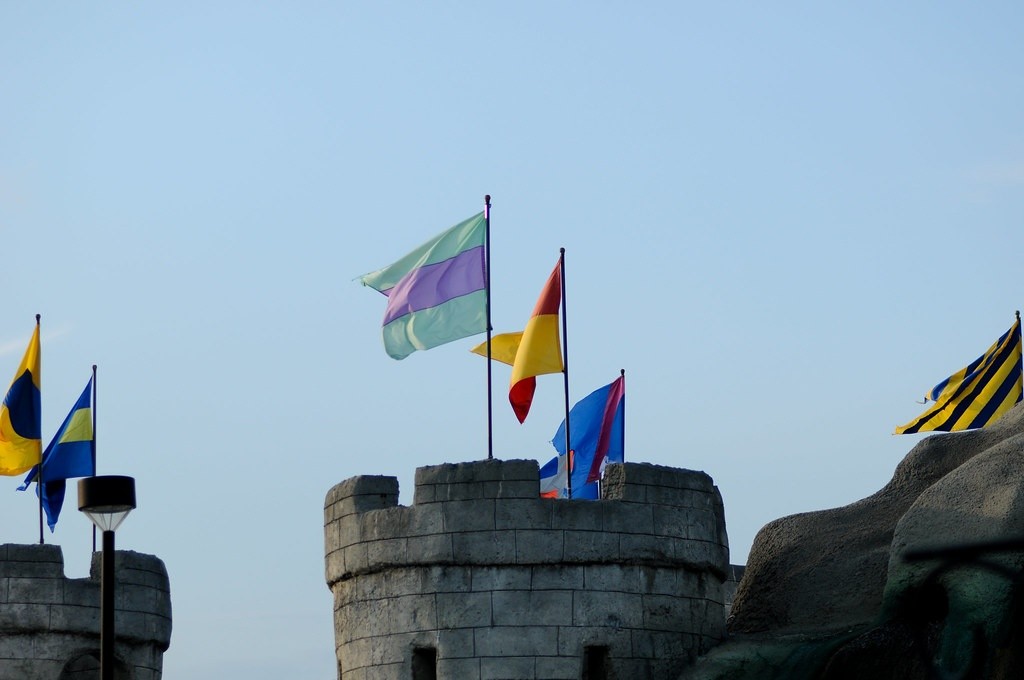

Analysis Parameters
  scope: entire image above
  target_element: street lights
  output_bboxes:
[77,475,136,680]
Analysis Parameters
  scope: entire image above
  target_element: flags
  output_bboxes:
[16,373,93,534]
[892,320,1023,435]
[360,211,489,360]
[0,321,42,476]
[540,376,625,499]
[469,258,562,424]
